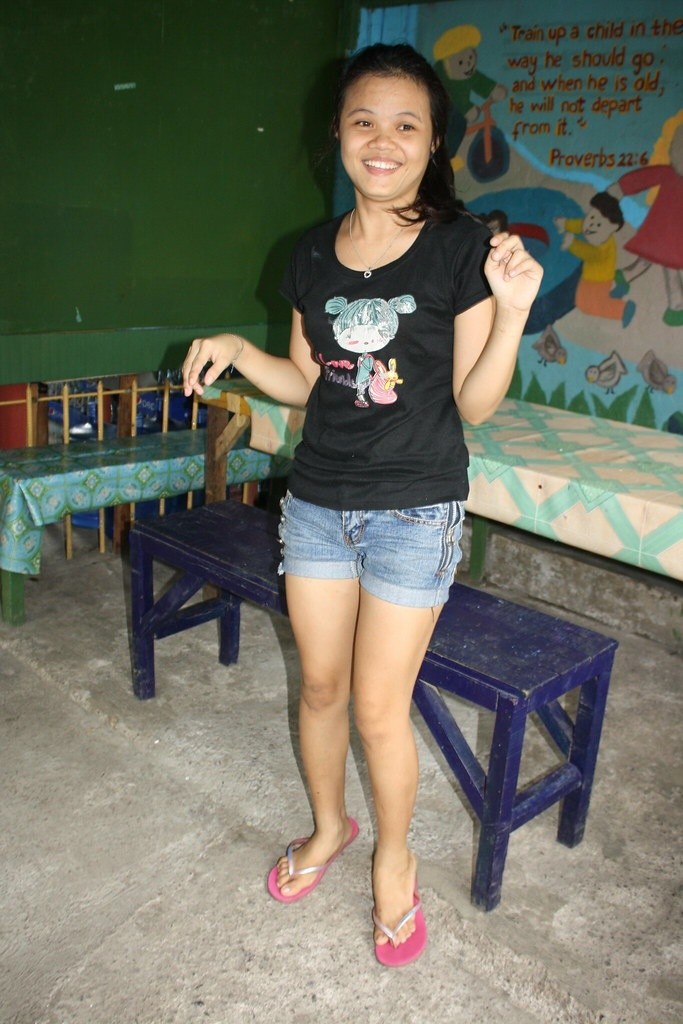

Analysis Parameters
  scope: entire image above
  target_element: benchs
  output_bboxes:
[0,426,294,625]
[125,509,619,911]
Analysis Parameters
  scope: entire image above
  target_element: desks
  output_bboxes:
[193,378,682,603]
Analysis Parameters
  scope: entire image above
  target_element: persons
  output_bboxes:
[181,43,545,965]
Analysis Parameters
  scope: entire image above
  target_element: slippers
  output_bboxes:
[268,816,359,904]
[372,874,427,968]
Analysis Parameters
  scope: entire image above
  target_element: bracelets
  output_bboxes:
[224,332,244,373]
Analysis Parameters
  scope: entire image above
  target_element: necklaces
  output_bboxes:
[349,208,412,279]
